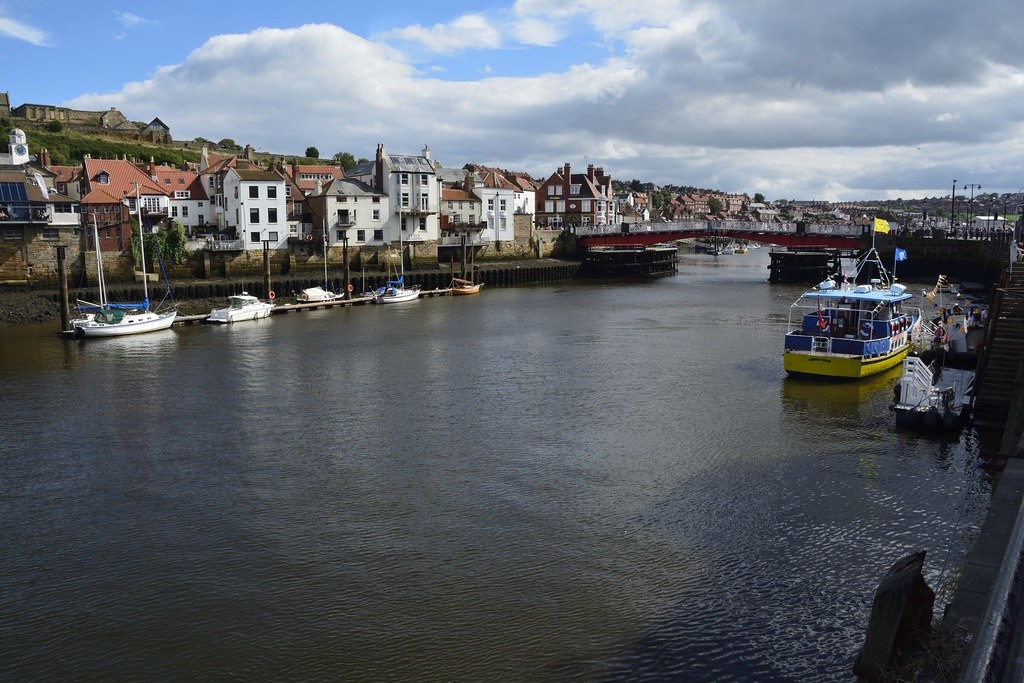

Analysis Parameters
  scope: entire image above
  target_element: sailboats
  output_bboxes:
[56,182,177,336]
[373,209,420,304]
[293,217,344,303]
[448,234,484,296]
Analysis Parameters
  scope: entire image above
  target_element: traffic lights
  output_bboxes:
[923,212,927,220]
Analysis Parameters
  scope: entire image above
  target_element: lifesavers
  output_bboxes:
[347,284,353,292]
[859,323,874,337]
[815,318,830,332]
[269,291,276,299]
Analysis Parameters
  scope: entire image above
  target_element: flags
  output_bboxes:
[896,248,907,261]
[819,302,825,328]
[874,218,890,234]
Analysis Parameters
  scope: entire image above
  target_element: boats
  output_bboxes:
[890,356,975,439]
[207,292,275,323]
[782,217,923,378]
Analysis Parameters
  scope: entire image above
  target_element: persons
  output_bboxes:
[970,227,1014,239]
[1016,250,1021,263]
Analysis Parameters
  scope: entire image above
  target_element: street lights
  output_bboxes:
[951,179,957,231]
[963,184,981,237]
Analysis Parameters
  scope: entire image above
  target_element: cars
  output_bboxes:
[735,249,749,254]
[706,246,718,255]
[722,246,735,255]
